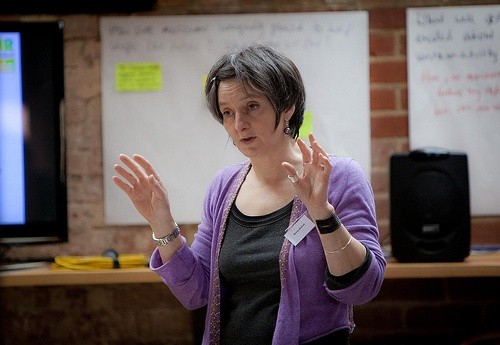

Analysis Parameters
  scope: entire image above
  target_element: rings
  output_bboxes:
[319,162,325,167]
[287,171,299,183]
[301,160,312,166]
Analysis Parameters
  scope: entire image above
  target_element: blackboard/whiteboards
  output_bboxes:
[406,5,500,218]
[100,10,372,228]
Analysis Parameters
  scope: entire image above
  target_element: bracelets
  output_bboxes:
[152,223,180,247]
[316,213,341,234]
[323,236,352,254]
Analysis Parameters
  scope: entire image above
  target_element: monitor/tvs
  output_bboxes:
[0,21,76,272]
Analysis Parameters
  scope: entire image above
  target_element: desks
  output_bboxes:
[0,249,500,345]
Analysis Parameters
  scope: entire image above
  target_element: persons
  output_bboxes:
[112,44,387,345]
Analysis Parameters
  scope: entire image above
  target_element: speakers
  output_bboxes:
[389,148,471,262]
